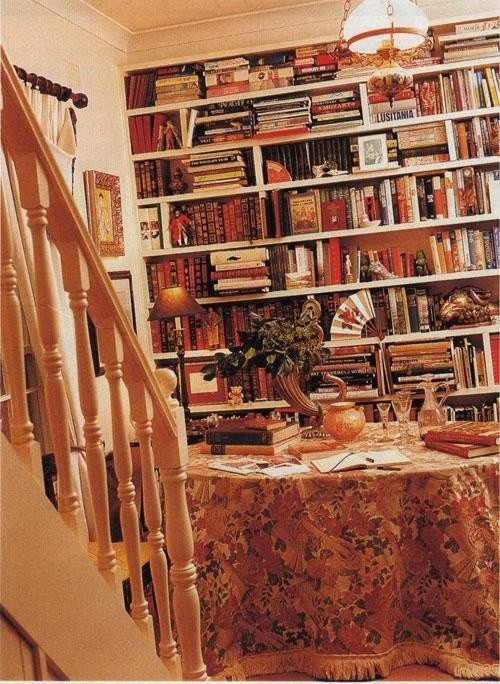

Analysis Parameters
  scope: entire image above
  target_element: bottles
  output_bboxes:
[323,401,366,444]
[417,373,450,440]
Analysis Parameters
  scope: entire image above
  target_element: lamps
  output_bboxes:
[335,0,435,110]
[144,284,207,443]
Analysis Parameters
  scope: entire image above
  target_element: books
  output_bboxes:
[126,20,500,109]
[184,332,498,478]
[146,225,500,355]
[128,67,500,250]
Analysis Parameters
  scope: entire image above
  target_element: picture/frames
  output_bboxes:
[84,269,138,379]
[84,169,128,258]
[285,191,320,235]
[355,133,391,171]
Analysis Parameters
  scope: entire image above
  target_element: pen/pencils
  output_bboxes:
[377,466,400,469]
[366,457,374,462]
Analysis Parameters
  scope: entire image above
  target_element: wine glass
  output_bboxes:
[390,391,414,450]
[376,402,394,443]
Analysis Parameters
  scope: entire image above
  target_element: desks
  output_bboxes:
[146,420,498,682]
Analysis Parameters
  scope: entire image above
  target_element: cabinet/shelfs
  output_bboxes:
[117,10,500,421]
